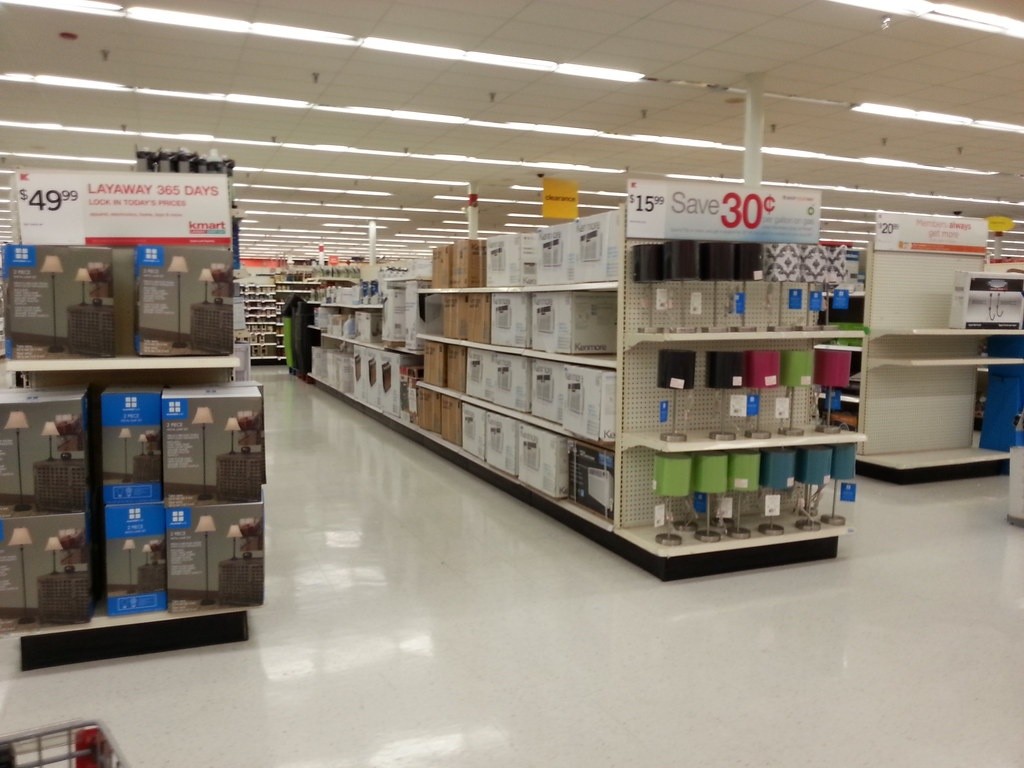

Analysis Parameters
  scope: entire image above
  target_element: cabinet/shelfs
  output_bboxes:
[238,273,433,442]
[313,211,617,521]
[813,240,1023,485]
[416,203,867,582]
[6,356,248,672]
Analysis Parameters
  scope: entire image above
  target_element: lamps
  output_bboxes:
[631,238,857,548]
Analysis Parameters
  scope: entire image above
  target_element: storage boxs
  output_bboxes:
[948,269,1024,330]
[0,246,270,636]
